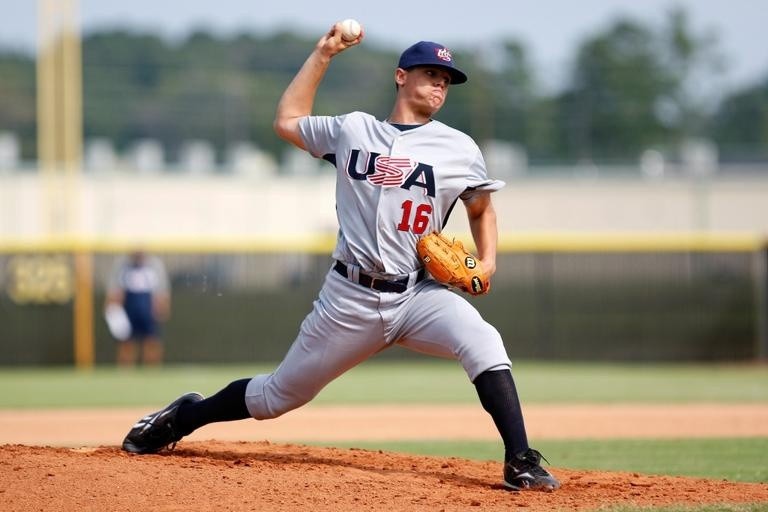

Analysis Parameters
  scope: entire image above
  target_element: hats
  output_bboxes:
[398,41,468,85]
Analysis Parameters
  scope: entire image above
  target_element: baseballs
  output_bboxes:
[339,19,361,41]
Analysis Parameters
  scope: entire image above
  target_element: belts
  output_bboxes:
[331,260,427,294]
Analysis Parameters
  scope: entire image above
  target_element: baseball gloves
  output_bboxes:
[416,231,490,296]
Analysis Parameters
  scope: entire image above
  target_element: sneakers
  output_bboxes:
[120,390,206,455]
[502,446,560,492]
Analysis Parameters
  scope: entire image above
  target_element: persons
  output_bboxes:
[101,252,170,367]
[122,21,558,492]
